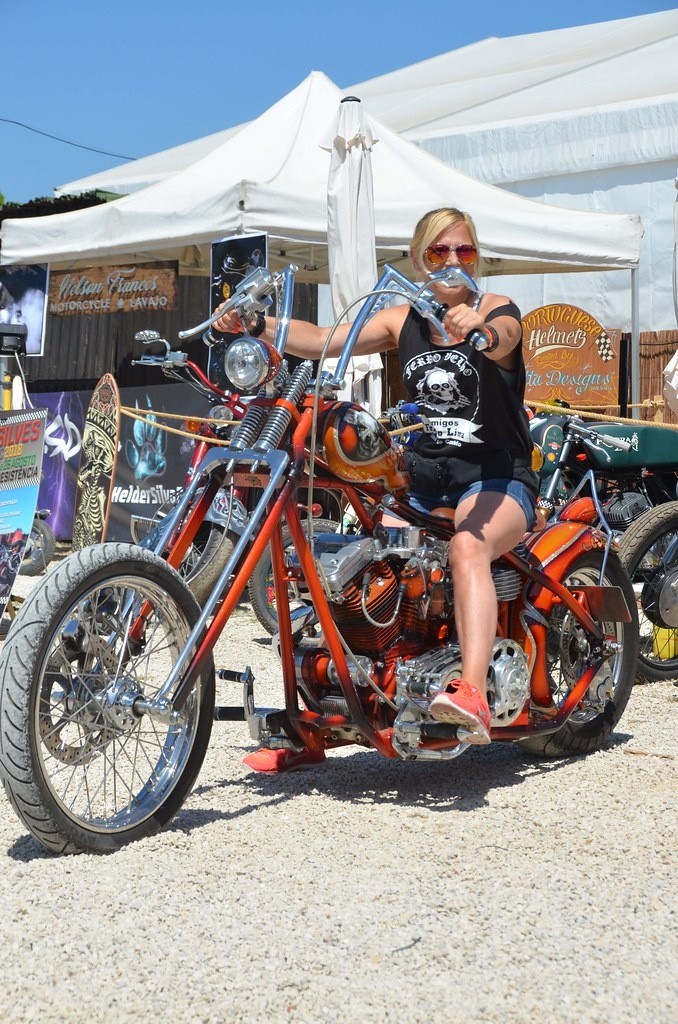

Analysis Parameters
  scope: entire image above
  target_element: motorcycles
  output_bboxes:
[1,263,678,857]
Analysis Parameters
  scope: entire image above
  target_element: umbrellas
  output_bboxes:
[324,95,384,433]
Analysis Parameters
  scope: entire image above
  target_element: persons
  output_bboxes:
[210,208,537,774]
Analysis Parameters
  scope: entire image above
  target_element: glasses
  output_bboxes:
[425,243,478,266]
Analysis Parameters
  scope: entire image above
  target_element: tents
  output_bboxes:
[0,5,677,543]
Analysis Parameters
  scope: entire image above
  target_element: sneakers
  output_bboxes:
[431,679,491,744]
[242,747,327,776]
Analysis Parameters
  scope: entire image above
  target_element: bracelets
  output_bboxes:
[249,314,266,338]
[482,323,499,352]
